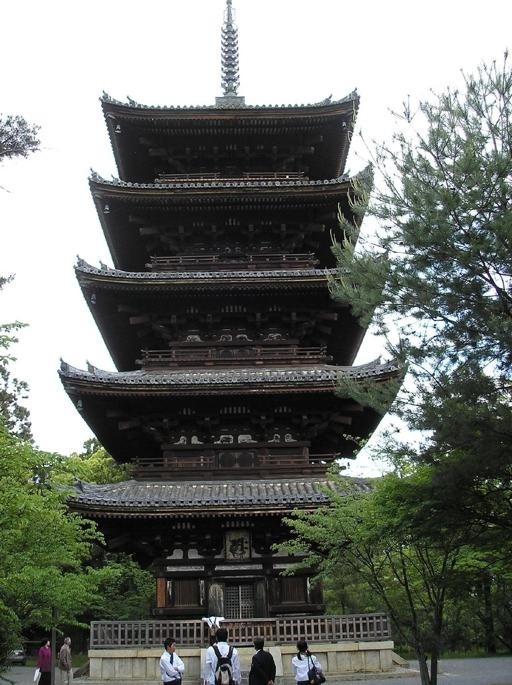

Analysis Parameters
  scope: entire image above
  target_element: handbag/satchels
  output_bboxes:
[307,654,326,684]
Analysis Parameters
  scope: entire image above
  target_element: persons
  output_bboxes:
[36,637,53,684]
[247,635,276,684]
[57,636,74,685]
[291,639,326,685]
[201,612,225,646]
[157,637,185,685]
[202,628,242,685]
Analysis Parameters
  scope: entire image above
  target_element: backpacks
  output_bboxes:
[212,645,234,684]
[209,616,218,637]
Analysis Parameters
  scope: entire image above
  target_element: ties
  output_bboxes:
[170,652,173,667]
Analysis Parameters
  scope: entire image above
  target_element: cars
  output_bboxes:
[0,639,27,665]
